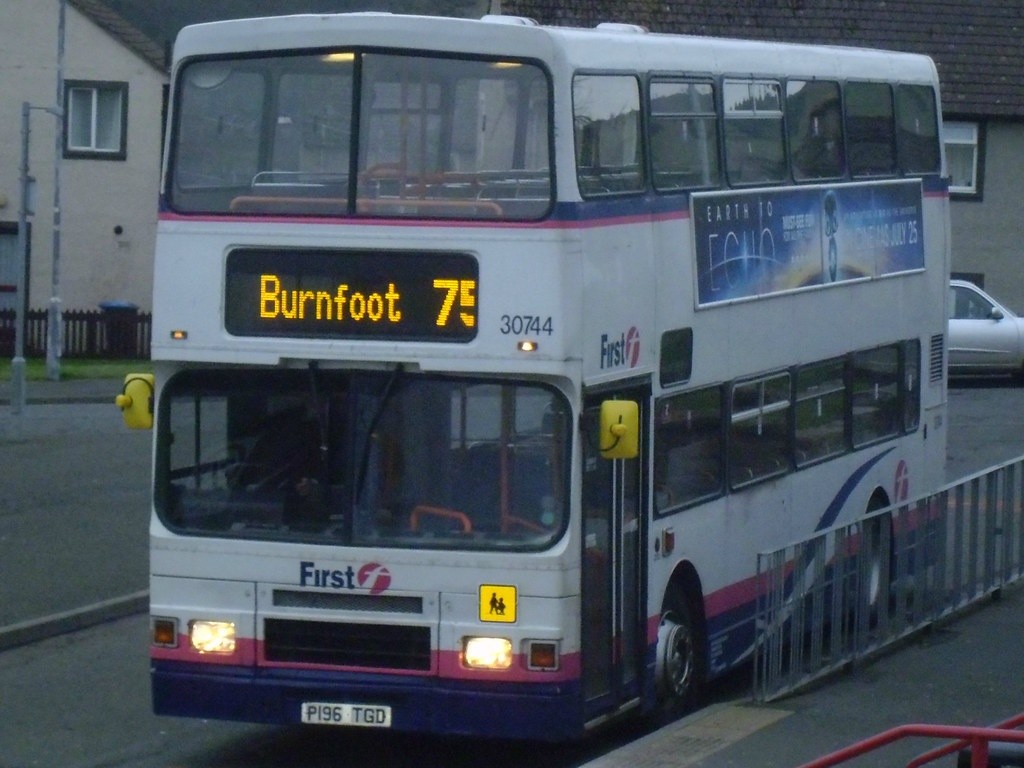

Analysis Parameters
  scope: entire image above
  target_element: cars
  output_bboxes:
[948,279,1024,387]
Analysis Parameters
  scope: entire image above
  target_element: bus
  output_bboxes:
[116,13,950,741]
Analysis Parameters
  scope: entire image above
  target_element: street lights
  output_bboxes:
[10,101,63,417]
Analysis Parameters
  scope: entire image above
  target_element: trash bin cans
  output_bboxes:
[97,301,139,359]
